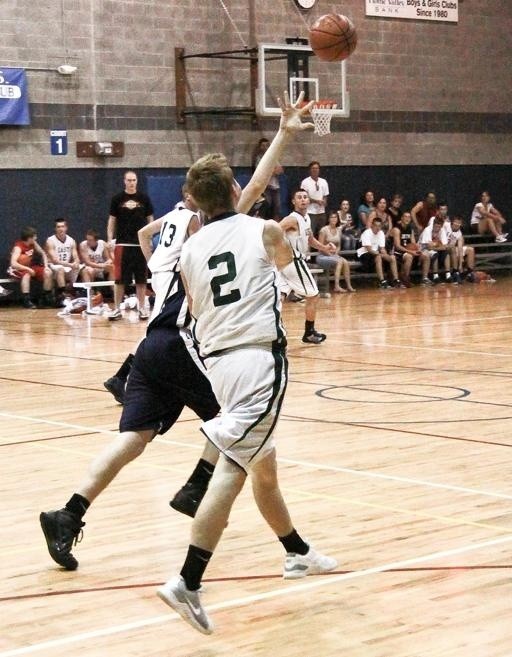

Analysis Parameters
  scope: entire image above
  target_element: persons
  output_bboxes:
[275,188,332,345]
[39,172,223,572]
[299,160,329,239]
[470,190,508,242]
[317,190,476,292]
[6,219,128,310]
[108,172,154,319]
[253,138,284,222]
[158,87,336,635]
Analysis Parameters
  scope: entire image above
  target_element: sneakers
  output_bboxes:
[495,233,508,242]
[157,573,214,635]
[378,268,464,288]
[320,292,331,298]
[302,330,326,343]
[108,309,122,318]
[139,307,148,318]
[104,376,128,404]
[346,286,356,292]
[169,482,229,528]
[40,508,85,570]
[284,541,338,579]
[334,287,346,292]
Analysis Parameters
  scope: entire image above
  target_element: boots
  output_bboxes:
[22,293,37,308]
[40,289,56,309]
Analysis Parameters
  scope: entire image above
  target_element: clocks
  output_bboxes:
[294,0,316,11]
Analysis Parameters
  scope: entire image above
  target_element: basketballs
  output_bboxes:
[309,12,357,61]
